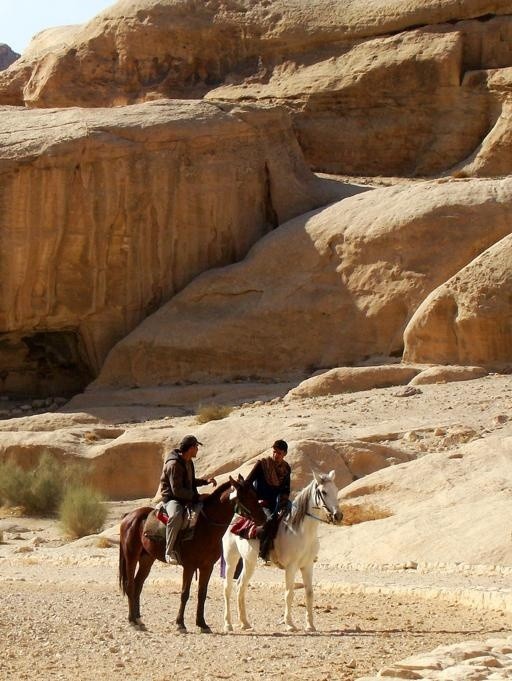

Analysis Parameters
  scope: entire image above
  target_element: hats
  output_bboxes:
[180,435,203,446]
[273,441,287,450]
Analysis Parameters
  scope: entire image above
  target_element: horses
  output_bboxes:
[118,473,267,633]
[221,469,343,632]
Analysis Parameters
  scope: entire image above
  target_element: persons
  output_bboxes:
[160,435,217,565]
[242,440,292,566]
